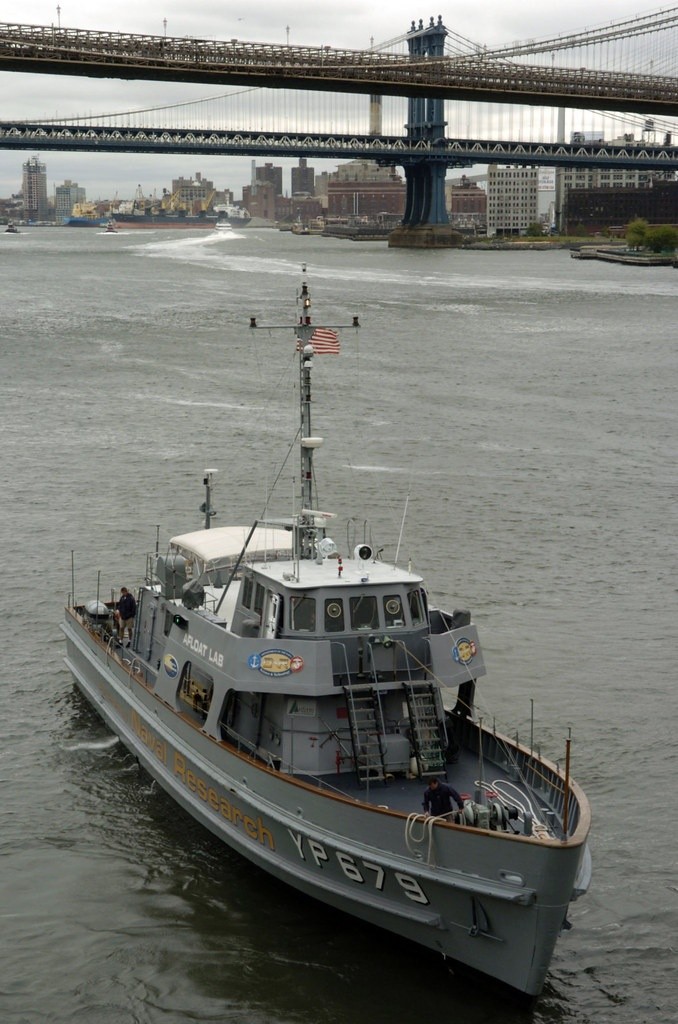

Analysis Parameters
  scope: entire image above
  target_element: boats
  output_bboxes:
[57,258,593,1023]
[61,199,110,228]
[109,187,253,231]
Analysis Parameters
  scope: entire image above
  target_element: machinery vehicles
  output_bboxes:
[134,183,217,218]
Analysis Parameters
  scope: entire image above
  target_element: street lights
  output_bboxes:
[370,36,374,52]
[285,25,290,44]
[162,17,167,43]
[56,4,62,33]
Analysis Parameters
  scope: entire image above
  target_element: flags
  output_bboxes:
[296,327,341,354]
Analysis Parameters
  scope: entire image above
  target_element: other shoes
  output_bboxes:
[125,642,131,648]
[115,642,124,648]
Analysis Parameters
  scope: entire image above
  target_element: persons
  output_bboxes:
[422,775,464,822]
[115,587,136,648]
